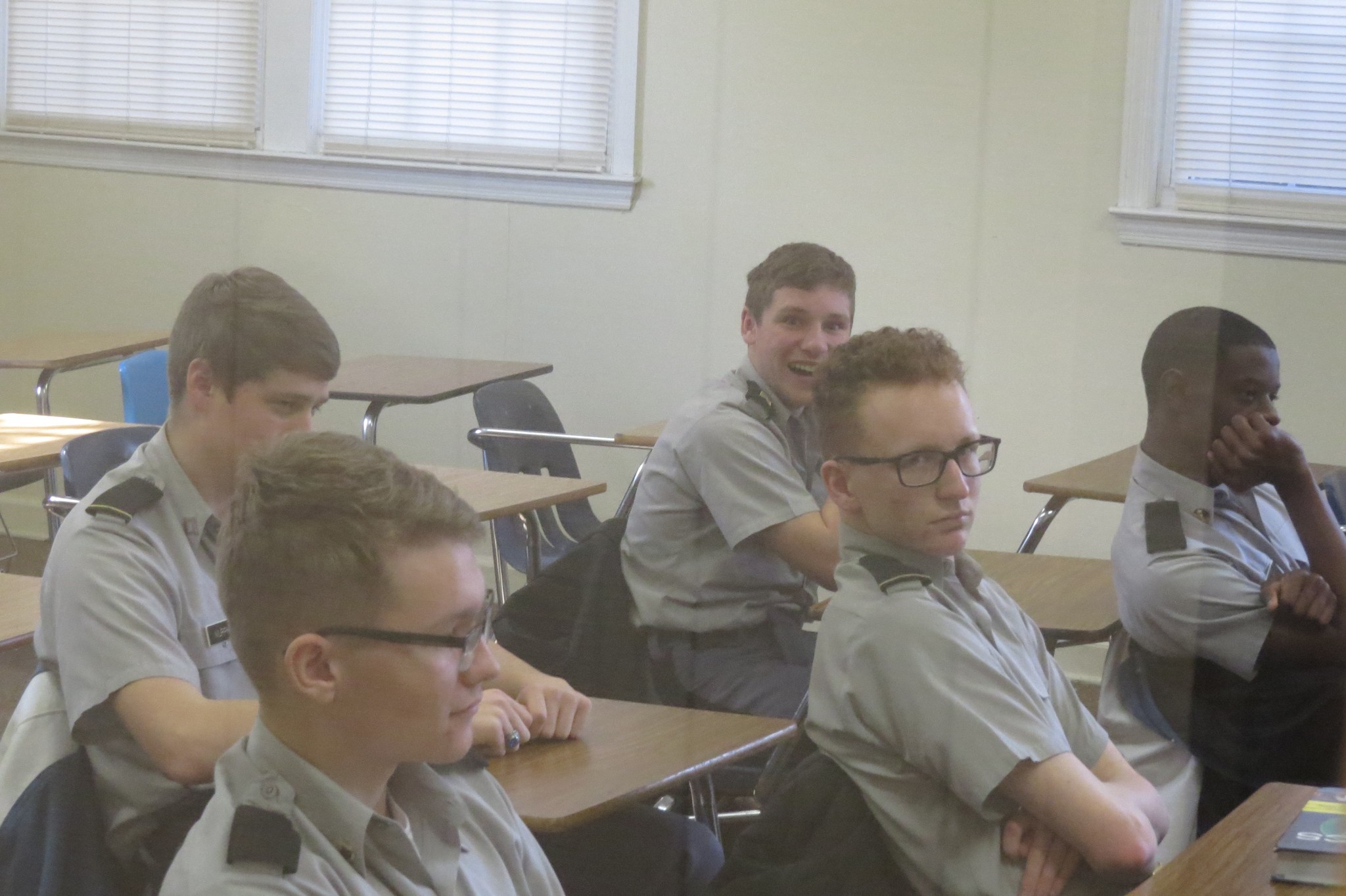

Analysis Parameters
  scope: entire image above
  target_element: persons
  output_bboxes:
[39,268,723,896]
[1111,306,1346,788]
[619,244,854,722]
[804,329,1171,896]
[159,432,566,896]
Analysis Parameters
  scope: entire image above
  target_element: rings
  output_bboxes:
[506,731,521,748]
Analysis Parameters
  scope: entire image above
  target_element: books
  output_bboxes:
[1272,787,1346,888]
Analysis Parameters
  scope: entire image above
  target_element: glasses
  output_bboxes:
[281,590,496,671]
[834,433,1001,488]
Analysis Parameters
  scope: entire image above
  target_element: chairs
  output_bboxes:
[1097,625,1257,864]
[467,377,601,575]
[0,658,138,896]
[42,426,162,521]
[118,349,170,425]
[559,448,667,705]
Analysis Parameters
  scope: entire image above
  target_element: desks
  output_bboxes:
[1122,780,1346,896]
[0,412,142,546]
[1015,443,1342,555]
[485,695,799,845]
[961,550,1122,662]
[0,328,170,416]
[0,572,43,652]
[325,353,557,451]
[416,462,609,607]
